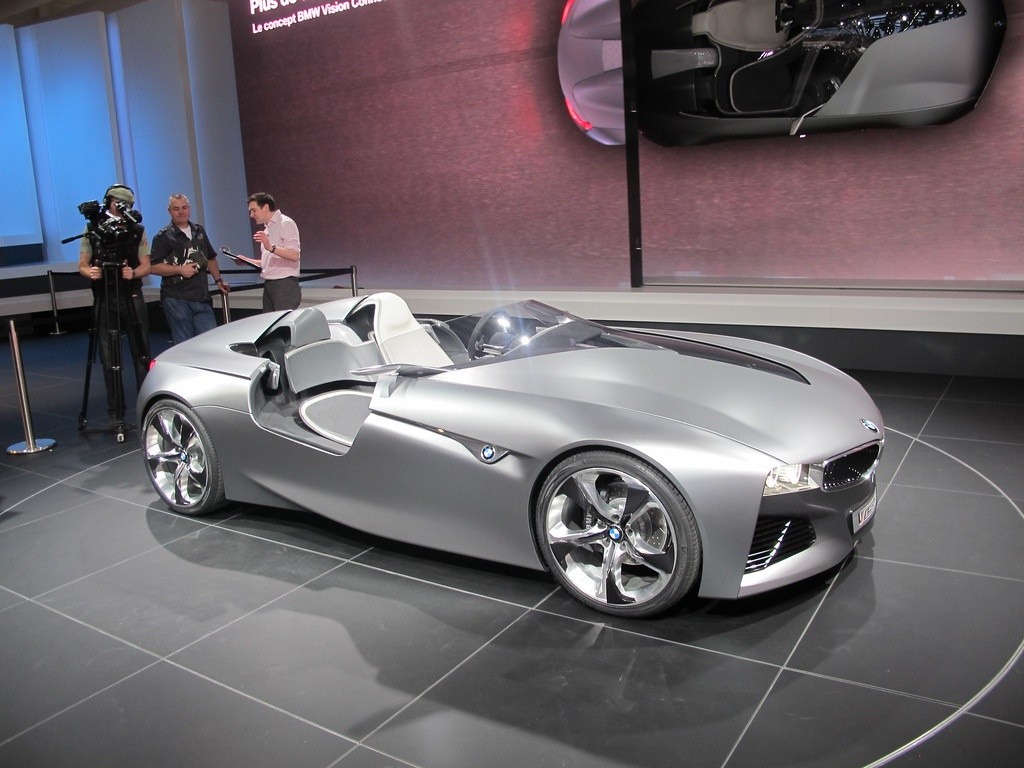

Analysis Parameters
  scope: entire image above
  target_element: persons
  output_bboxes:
[233,192,302,312]
[149,194,230,345]
[79,184,151,433]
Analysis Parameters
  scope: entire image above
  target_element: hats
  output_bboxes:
[105,184,135,203]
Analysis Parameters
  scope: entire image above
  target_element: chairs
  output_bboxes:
[371,291,454,369]
[286,306,372,445]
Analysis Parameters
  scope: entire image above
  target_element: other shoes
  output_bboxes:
[112,418,127,435]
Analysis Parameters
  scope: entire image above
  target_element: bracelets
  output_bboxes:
[271,244,275,253]
[133,270,135,278]
[216,278,223,283]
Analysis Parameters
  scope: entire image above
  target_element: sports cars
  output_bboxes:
[133,289,887,619]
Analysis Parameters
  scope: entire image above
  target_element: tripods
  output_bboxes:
[76,256,151,443]
